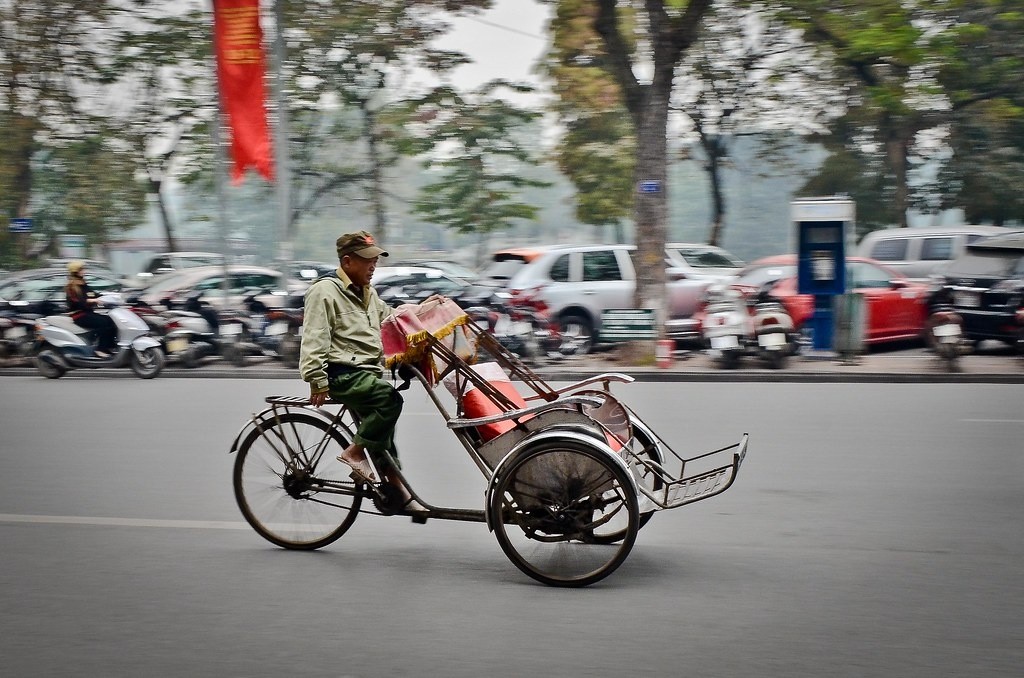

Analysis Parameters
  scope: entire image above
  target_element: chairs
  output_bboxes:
[442,358,633,478]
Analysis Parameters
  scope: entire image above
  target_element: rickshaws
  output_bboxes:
[229,293,749,587]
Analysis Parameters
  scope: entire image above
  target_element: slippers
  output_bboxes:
[403,499,431,512]
[335,454,377,482]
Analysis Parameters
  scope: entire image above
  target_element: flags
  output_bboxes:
[212,1,274,185]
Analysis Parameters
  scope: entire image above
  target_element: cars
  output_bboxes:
[942,230,1024,348]
[477,241,743,355]
[1,251,503,310]
[694,253,936,352]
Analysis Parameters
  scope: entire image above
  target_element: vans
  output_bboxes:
[857,225,1012,282]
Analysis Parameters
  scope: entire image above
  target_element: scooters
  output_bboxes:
[920,278,964,368]
[750,279,800,371]
[702,289,753,367]
[126,268,320,368]
[0,300,68,366]
[34,294,162,380]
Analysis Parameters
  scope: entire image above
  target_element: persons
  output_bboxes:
[298,230,448,513]
[65,261,117,358]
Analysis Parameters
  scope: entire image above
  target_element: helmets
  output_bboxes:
[68,262,85,278]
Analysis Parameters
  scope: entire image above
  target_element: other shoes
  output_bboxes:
[92,348,118,359]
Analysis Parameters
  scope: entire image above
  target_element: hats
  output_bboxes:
[336,231,389,261]
